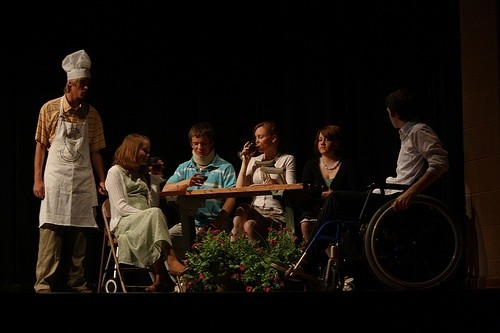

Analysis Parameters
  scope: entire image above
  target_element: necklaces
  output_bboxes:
[320,154,343,171]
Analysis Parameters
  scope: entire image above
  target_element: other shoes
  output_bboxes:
[79,289,93,293]
[37,288,52,292]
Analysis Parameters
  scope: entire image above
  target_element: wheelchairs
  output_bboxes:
[280,127,468,293]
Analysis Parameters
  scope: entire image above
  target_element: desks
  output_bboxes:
[157,182,324,262]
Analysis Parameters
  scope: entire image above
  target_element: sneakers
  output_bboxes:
[271,258,322,278]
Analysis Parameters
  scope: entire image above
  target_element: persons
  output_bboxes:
[297,125,361,249]
[32,49,107,296]
[163,124,240,256]
[229,121,299,250]
[105,134,197,294]
[331,89,447,269]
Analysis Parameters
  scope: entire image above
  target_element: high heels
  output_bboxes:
[168,265,190,279]
[146,282,163,292]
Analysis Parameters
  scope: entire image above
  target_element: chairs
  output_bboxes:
[285,162,463,290]
[98,198,164,293]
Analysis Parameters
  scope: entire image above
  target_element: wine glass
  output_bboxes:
[237,141,256,159]
[195,168,205,190]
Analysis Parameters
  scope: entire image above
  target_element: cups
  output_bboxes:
[151,157,161,174]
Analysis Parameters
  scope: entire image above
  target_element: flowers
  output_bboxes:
[171,220,311,296]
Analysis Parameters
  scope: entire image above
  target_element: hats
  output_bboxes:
[62,49,92,80]
